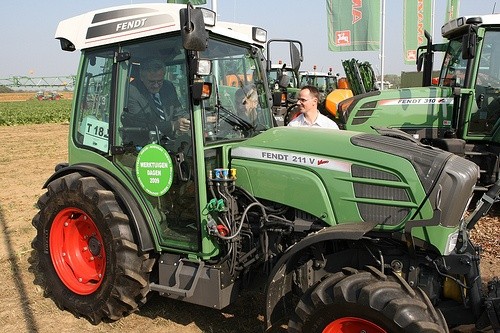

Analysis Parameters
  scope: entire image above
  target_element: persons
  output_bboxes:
[286,85,340,130]
[213,81,269,141]
[123,55,216,229]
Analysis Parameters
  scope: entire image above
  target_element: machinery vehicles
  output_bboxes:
[30,1,500,333]
[336,12,500,196]
[268,63,346,128]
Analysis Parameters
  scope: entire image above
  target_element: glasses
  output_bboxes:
[144,72,165,83]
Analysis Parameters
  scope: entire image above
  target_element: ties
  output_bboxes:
[152,94,165,120]
